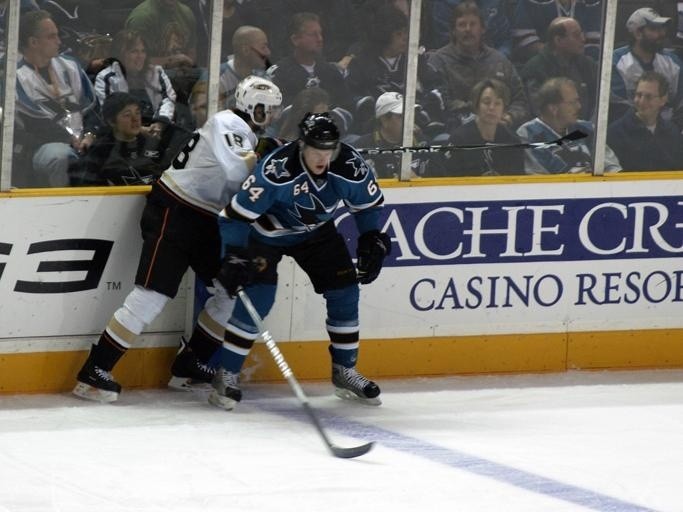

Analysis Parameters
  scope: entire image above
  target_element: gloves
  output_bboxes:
[356,229,391,284]
[217,245,265,299]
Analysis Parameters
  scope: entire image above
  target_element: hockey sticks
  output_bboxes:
[356,130,588,154]
[237,285,375,458]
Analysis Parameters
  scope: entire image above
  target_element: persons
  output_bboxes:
[207,112,391,412]
[72,74,283,403]
[1,1,682,189]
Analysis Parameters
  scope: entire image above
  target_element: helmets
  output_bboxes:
[234,74,283,125]
[298,112,340,150]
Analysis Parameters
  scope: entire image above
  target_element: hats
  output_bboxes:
[625,7,672,33]
[375,91,423,120]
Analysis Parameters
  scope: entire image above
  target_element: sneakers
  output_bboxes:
[211,366,242,402]
[76,343,121,394]
[332,361,380,399]
[171,335,218,383]
